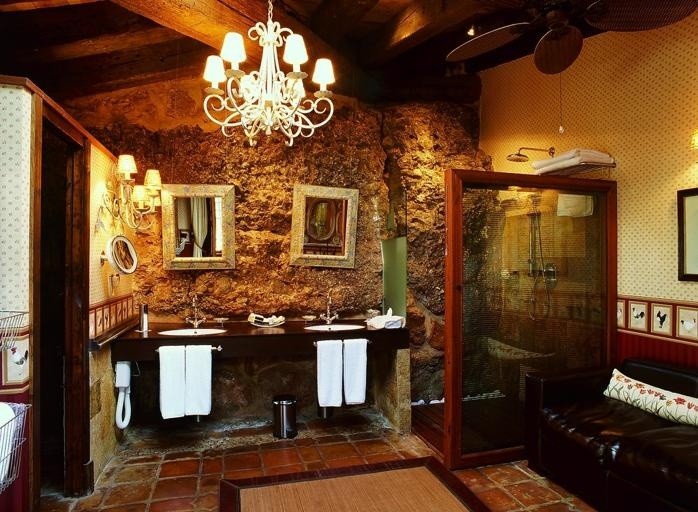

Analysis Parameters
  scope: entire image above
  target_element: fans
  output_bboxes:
[444,0,696,78]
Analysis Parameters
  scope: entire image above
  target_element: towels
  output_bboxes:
[157,344,213,420]
[315,338,369,408]
[367,307,406,329]
[531,148,614,175]
[557,193,594,218]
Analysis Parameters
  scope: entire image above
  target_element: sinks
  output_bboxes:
[157,328,229,335]
[303,324,366,332]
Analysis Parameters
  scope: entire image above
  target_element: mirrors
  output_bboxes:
[100,235,138,275]
[676,187,698,282]
[289,181,359,270]
[159,183,237,272]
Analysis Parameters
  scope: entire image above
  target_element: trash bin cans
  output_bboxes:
[271,394,298,440]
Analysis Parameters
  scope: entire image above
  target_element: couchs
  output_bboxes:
[522,357,698,510]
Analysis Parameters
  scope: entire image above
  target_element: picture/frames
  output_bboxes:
[614,300,698,343]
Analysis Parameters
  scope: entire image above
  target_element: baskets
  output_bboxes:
[0,310,29,352]
[0,401,31,492]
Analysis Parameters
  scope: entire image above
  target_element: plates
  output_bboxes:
[250,321,286,329]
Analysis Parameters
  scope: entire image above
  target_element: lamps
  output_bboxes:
[103,153,162,230]
[202,1,340,148]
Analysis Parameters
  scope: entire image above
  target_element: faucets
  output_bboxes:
[320,296,339,325]
[185,298,206,329]
